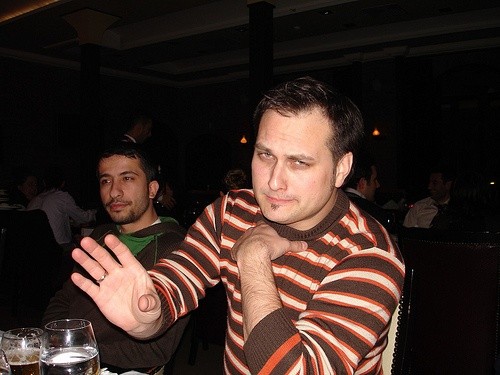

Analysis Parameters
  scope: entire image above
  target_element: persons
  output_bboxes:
[344,158,400,241]
[71,77,405,375]
[42,146,194,374]
[403,163,482,229]
[14,111,252,273]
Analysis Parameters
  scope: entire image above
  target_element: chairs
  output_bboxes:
[0,205,500,375]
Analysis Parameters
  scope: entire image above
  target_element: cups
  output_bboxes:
[40,318,100,375]
[2,327,44,375]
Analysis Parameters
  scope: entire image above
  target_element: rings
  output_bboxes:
[97,273,106,284]
